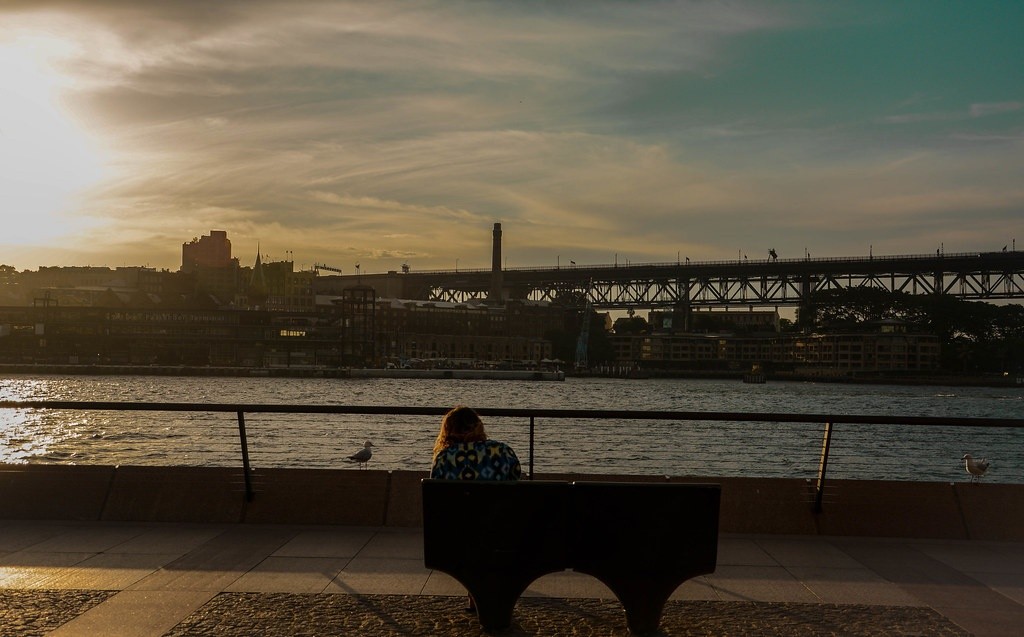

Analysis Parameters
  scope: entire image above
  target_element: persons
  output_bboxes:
[430,406,521,611]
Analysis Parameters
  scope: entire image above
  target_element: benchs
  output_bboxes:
[421,478,722,636]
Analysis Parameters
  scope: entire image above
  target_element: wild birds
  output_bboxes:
[961,454,990,482]
[346,440,374,470]
